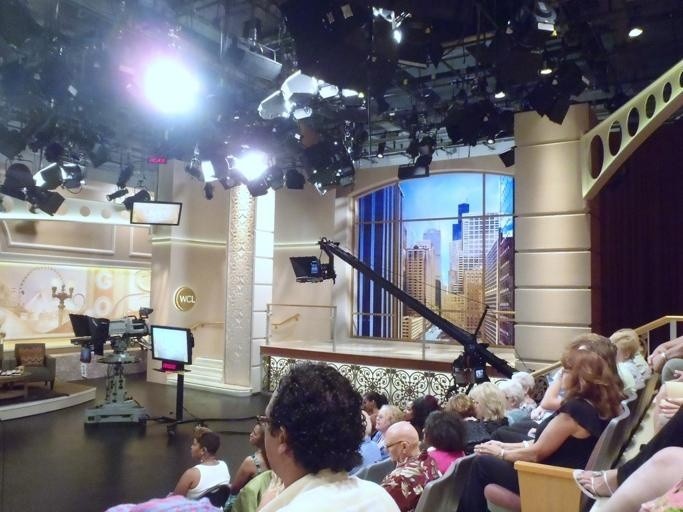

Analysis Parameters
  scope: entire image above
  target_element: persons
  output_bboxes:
[382,328,682,511]
[227,361,401,512]
[171,426,230,501]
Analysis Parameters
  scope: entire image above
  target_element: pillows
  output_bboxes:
[14,341,45,370]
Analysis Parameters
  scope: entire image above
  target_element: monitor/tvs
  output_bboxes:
[287,256,323,284]
[130,200,182,226]
[151,325,194,365]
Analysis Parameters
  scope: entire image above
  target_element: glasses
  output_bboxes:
[254,415,282,428]
[560,369,571,378]
[383,441,408,449]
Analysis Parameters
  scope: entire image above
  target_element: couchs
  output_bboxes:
[0,352,57,395]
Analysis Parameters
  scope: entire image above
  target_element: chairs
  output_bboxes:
[193,483,231,509]
[350,368,659,512]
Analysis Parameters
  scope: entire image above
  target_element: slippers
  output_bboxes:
[572,469,612,501]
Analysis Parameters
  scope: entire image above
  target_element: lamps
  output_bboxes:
[0,0,645,227]
